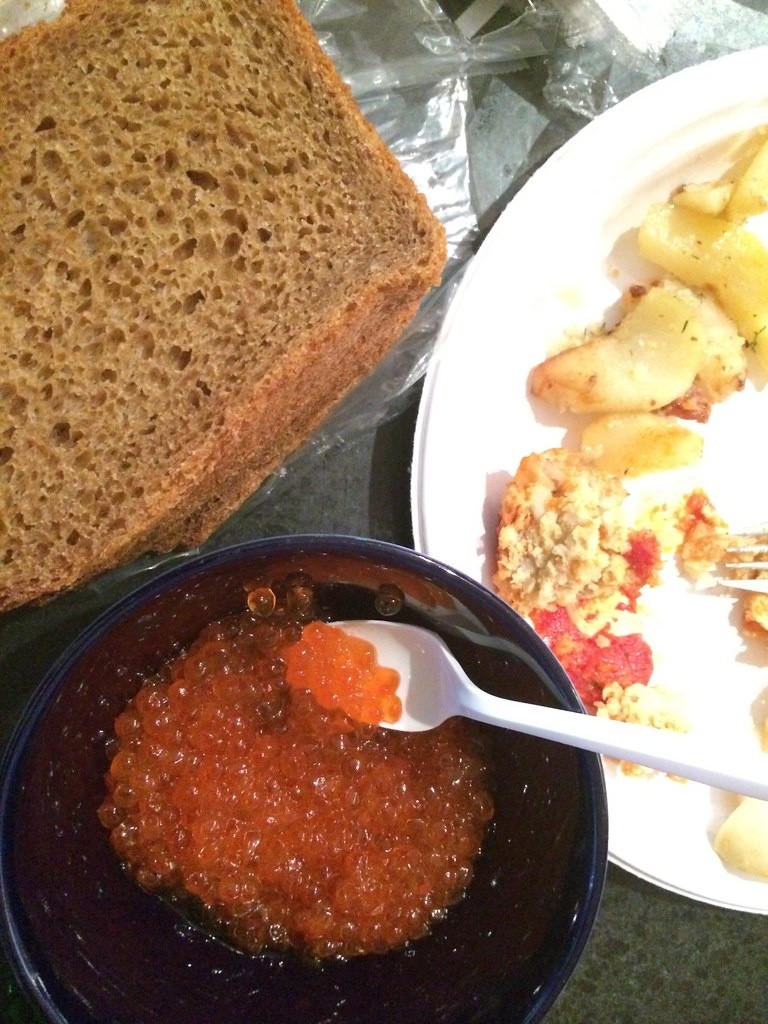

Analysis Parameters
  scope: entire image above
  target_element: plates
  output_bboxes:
[411,46,768,916]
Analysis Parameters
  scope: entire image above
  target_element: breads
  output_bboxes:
[1,1,445,615]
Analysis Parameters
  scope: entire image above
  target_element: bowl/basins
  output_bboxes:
[0,533,608,1024]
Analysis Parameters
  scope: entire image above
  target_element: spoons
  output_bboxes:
[326,621,768,800]
